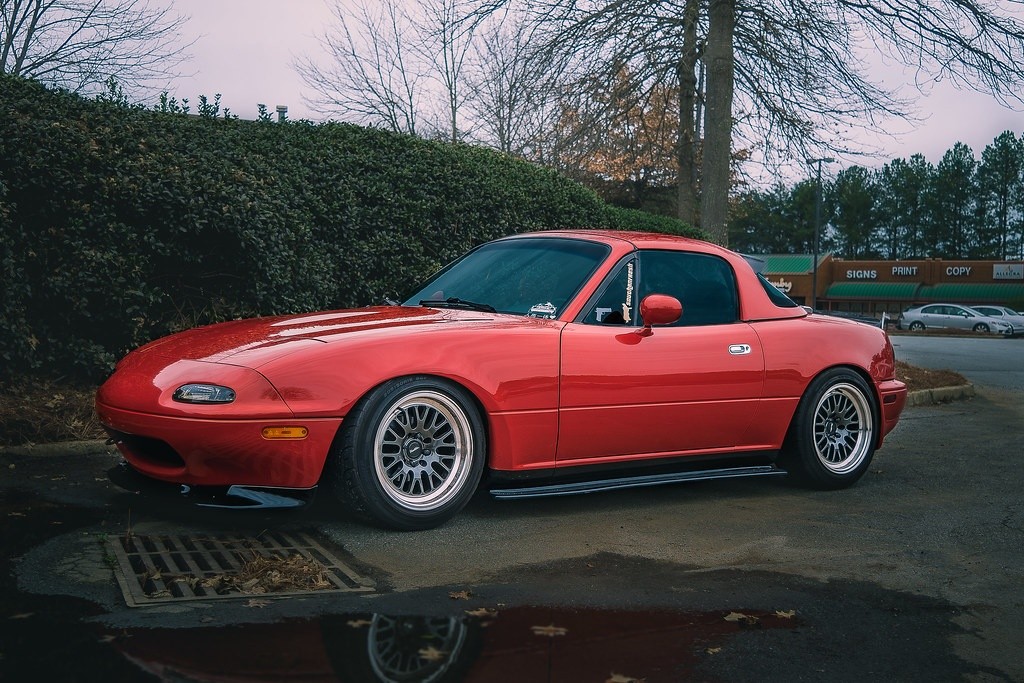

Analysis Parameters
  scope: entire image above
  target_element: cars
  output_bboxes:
[899,302,1014,338]
[957,305,1024,336]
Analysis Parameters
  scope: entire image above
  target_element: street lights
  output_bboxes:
[805,157,835,314]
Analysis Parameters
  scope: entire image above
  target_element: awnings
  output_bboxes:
[822,281,1024,305]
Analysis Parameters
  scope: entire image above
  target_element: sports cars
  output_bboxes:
[94,228,909,535]
